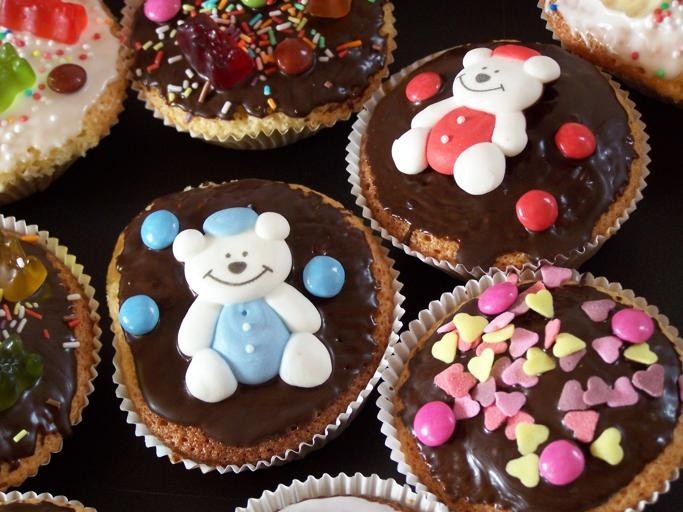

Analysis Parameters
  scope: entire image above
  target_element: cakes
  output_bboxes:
[1,0,683,512]
